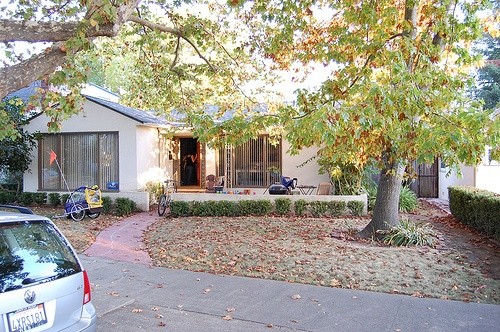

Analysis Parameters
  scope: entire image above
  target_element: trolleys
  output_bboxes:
[52,185,104,222]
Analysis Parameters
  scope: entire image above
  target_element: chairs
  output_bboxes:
[281,177,297,195]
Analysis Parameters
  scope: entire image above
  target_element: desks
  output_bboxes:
[297,186,316,195]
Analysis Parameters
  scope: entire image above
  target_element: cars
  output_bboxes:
[0,204,98,332]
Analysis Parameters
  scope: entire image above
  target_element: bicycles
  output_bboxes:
[157,179,180,216]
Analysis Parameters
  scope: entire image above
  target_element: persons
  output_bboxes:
[182,150,197,186]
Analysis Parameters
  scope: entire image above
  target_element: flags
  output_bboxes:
[49,152,57,164]
